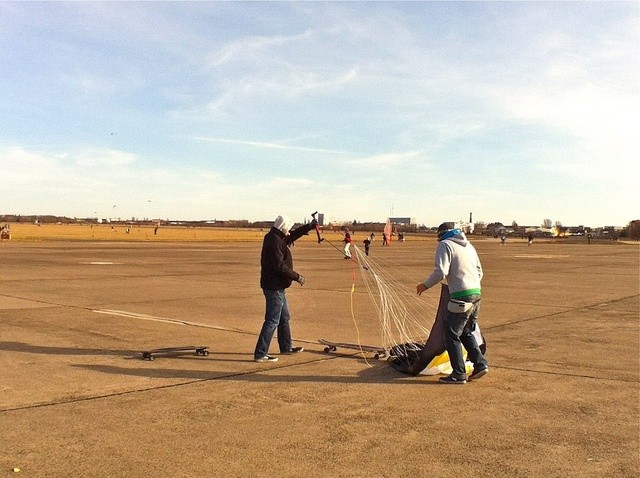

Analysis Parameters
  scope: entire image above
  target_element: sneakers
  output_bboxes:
[438,373,467,384]
[280,347,303,355]
[468,365,489,380]
[254,354,278,362]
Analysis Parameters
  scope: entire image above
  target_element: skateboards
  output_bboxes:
[318,339,391,359]
[140,346,209,361]
[344,257,351,260]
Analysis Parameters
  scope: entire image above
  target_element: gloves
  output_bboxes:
[297,275,305,286]
[309,219,317,230]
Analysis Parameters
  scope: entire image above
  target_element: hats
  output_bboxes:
[274,216,294,236]
[436,222,454,239]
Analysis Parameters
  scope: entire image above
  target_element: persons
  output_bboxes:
[382,230,388,246]
[363,238,370,256]
[527,234,533,247]
[342,227,352,259]
[501,233,506,244]
[254,216,319,362]
[585,231,593,244]
[417,223,489,384]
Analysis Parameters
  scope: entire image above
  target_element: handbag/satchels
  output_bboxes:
[447,297,474,314]
